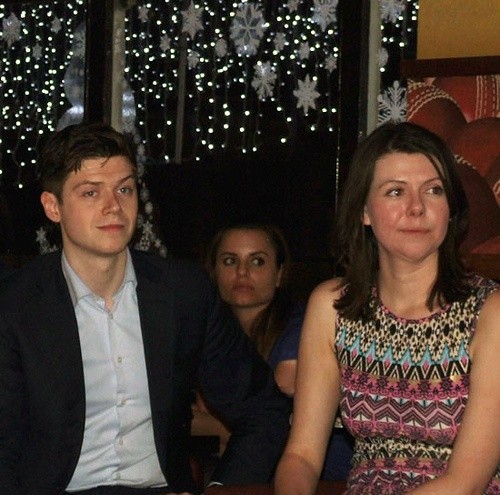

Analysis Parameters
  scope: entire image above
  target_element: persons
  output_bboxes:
[0,122,288,494]
[270,301,356,482]
[274,121,500,494]
[195,209,300,457]
